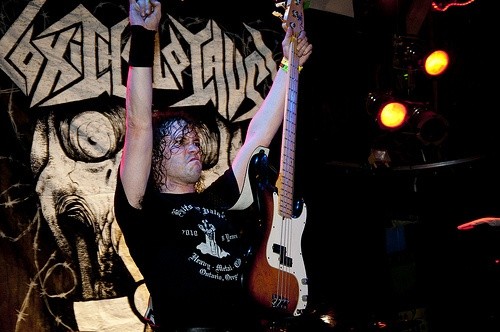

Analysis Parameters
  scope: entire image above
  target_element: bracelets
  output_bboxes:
[277,57,303,75]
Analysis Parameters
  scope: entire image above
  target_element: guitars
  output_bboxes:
[240,0,311,320]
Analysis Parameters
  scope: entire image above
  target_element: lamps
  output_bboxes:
[397,98,448,148]
[364,86,407,131]
[368,147,397,180]
[392,33,451,78]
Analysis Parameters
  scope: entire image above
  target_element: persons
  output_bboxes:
[115,0,314,332]
